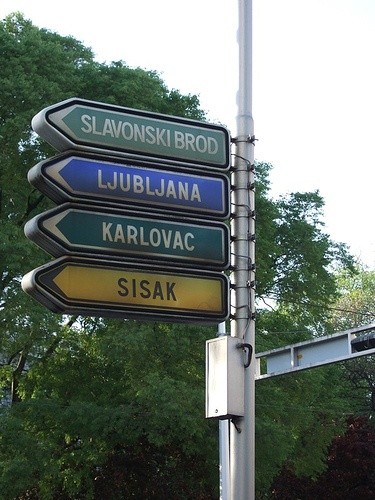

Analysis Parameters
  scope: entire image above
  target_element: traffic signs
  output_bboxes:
[21,256,230,322]
[28,150,231,221]
[31,97,232,174]
[23,203,231,271]
